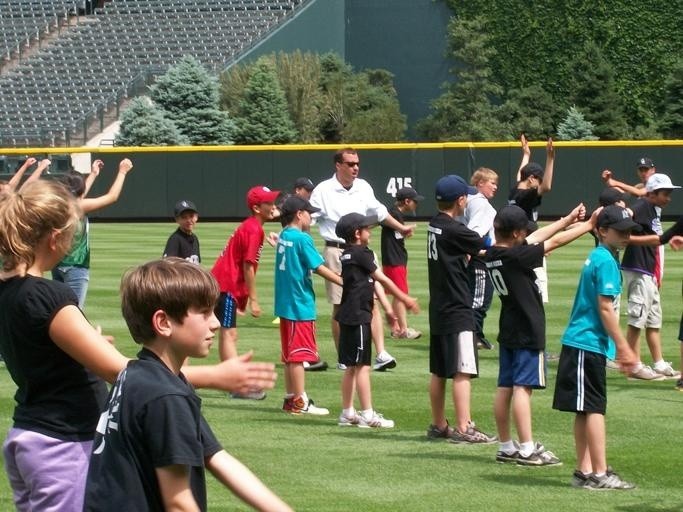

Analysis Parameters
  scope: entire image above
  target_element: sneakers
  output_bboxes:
[572,468,621,486]
[357,412,394,429]
[650,363,681,381]
[282,396,295,411]
[626,365,665,383]
[450,422,500,445]
[515,443,565,469]
[398,328,421,340]
[426,419,455,438]
[337,362,347,371]
[495,450,520,464]
[291,392,330,416]
[337,413,361,427]
[582,473,636,491]
[373,349,397,371]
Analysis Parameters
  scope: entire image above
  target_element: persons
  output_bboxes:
[426,168,498,444]
[267,147,426,427]
[603,157,682,390]
[0,158,51,201]
[550,206,639,490]
[82,254,297,512]
[206,186,281,400]
[162,201,200,267]
[0,177,276,511]
[50,157,132,309]
[485,134,603,464]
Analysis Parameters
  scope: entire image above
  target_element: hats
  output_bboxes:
[634,157,654,170]
[645,172,682,193]
[597,205,643,233]
[294,176,314,190]
[598,186,634,206]
[435,174,477,202]
[335,213,379,239]
[246,185,280,210]
[396,187,424,202]
[281,196,320,217]
[522,162,544,179]
[494,204,538,232]
[173,199,199,216]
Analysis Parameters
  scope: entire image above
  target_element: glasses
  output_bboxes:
[344,161,359,167]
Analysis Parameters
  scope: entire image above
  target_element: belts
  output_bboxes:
[325,240,346,249]
